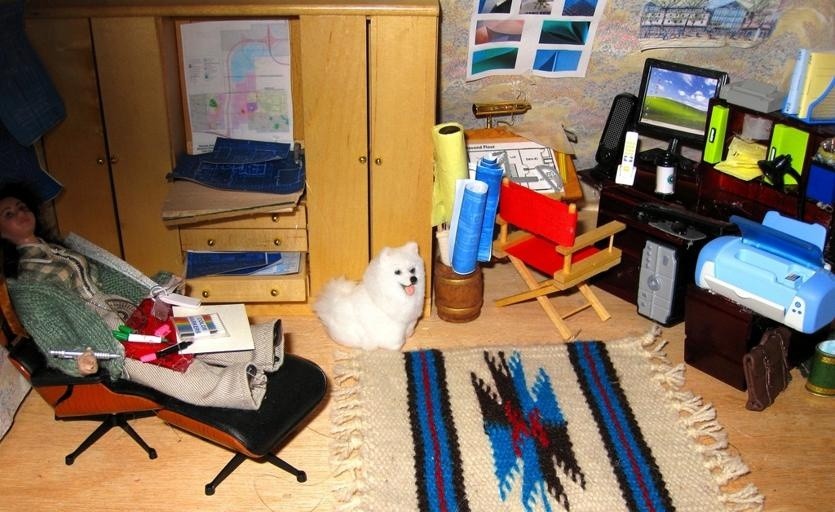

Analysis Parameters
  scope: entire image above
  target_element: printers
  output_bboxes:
[695,211,835,334]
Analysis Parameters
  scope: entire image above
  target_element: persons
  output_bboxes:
[0,177,285,410]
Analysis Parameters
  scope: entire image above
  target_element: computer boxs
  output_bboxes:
[636,216,708,328]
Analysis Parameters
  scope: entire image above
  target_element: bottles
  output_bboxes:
[656,149,676,197]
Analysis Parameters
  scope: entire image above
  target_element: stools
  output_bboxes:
[155,349,330,496]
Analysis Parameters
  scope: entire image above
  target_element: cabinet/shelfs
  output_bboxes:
[178,197,309,307]
[570,161,835,392]
[25,18,185,282]
[299,12,439,322]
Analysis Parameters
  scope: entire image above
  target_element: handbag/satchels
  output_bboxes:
[742,327,792,411]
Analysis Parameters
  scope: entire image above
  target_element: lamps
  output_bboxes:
[755,153,807,222]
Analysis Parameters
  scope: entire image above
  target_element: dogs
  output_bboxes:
[310,240,426,351]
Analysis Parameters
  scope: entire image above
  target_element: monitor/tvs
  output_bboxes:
[633,57,729,182]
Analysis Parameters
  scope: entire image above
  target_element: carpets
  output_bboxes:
[328,323,765,511]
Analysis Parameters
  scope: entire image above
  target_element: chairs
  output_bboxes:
[494,173,628,343]
[1,265,164,465]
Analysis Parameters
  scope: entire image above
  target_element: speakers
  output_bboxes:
[591,93,637,180]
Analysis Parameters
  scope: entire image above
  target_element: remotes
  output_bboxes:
[620,132,639,177]
